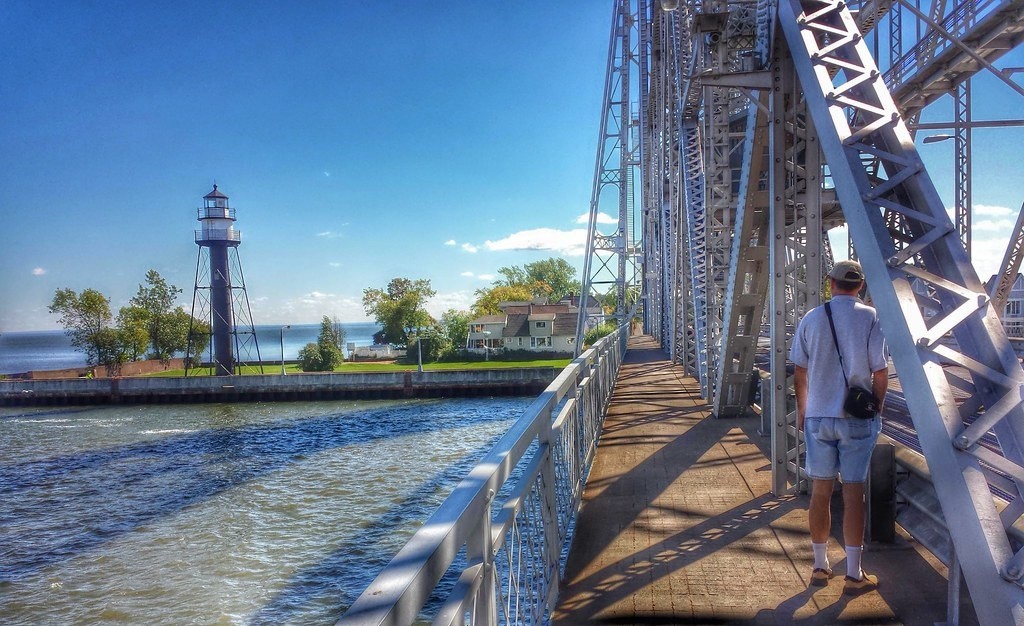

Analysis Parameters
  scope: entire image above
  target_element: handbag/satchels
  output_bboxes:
[844,385,881,422]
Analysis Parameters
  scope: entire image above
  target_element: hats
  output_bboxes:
[825,262,863,283]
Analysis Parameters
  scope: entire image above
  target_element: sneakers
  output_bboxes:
[843,569,881,596]
[810,567,833,587]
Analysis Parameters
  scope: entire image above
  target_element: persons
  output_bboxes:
[789,260,889,594]
